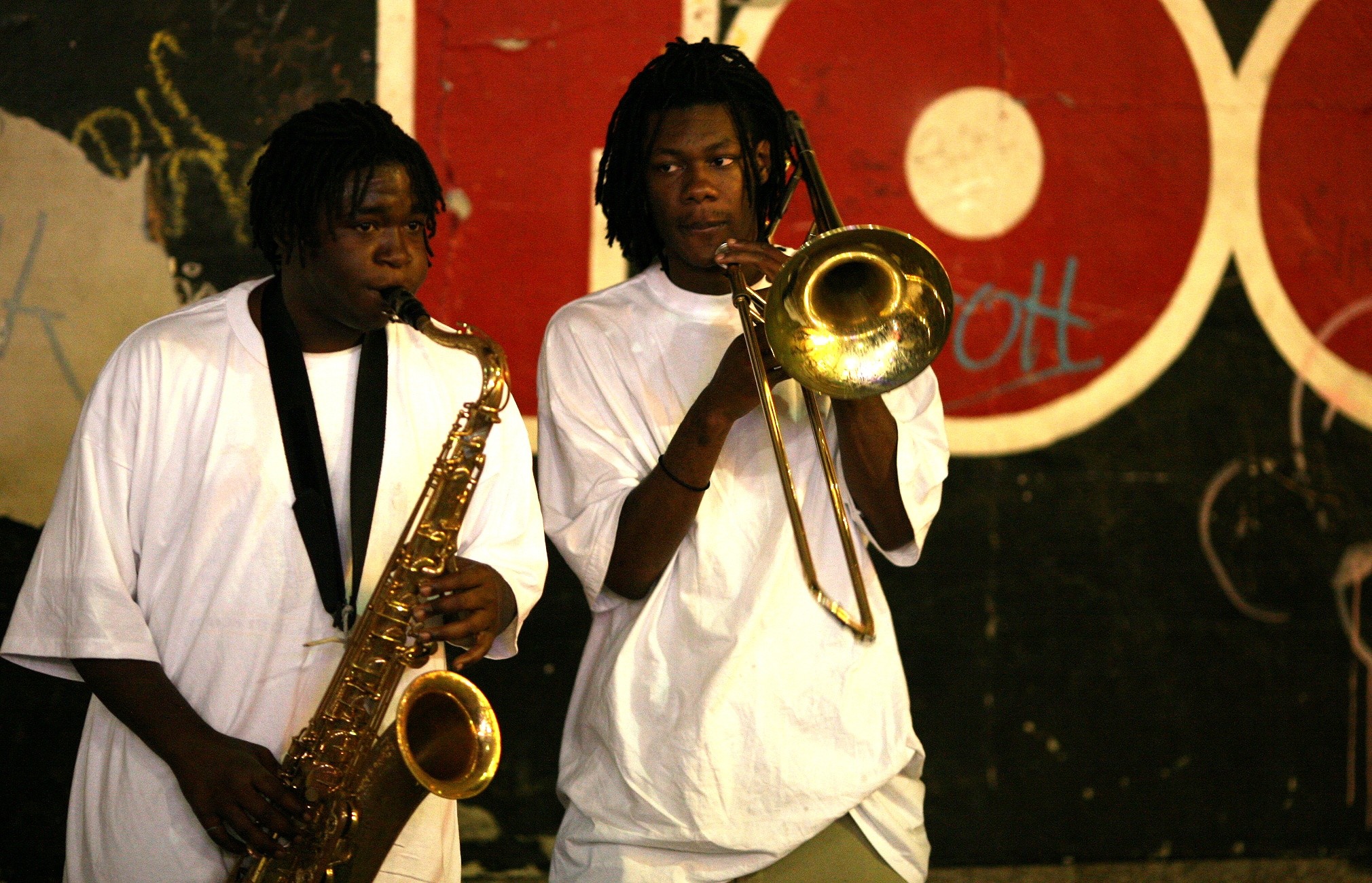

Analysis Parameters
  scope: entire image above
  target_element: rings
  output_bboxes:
[206,823,225,834]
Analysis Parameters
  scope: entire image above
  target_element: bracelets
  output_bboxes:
[653,449,714,493]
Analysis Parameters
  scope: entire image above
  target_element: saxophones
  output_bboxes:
[226,281,508,883]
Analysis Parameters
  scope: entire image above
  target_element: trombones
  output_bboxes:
[716,223,956,643]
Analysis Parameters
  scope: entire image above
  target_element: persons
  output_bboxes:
[0,97,550,883]
[533,34,975,883]
[5,117,191,636]
